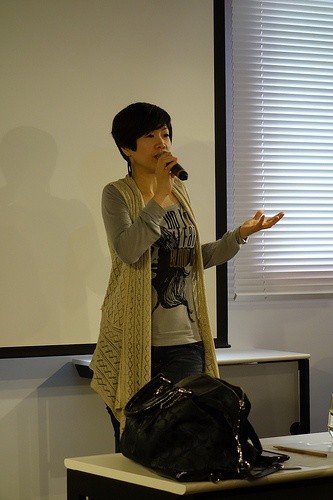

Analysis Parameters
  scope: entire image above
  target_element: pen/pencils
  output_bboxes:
[272,444,328,457]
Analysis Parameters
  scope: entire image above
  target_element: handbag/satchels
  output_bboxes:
[118,365,285,482]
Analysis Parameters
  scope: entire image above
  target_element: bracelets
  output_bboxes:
[241,236,249,244]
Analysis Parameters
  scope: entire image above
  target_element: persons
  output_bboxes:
[88,101,286,454]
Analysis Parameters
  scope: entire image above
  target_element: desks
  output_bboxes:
[64,430,333,500]
[71,348,312,436]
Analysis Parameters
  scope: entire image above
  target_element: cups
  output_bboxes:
[328,392,333,438]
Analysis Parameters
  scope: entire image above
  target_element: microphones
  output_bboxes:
[157,151,189,181]
